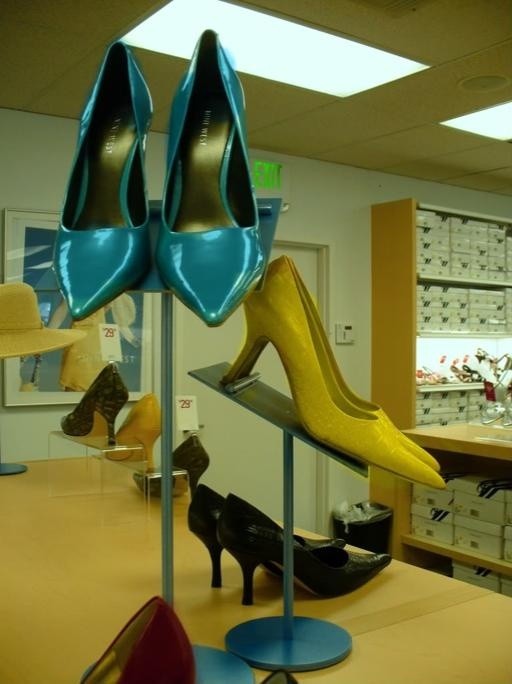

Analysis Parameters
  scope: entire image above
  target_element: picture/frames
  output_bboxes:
[2,208,156,408]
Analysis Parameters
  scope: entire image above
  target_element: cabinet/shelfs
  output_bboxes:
[371,200,512,585]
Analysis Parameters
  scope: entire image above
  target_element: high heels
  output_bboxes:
[60,363,129,447]
[186,484,347,587]
[105,393,162,473]
[256,667,300,683]
[132,433,211,502]
[216,493,392,605]
[219,254,448,492]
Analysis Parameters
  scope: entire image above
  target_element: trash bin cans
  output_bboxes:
[331,499,394,556]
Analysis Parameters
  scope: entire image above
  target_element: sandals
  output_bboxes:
[501,398,512,427]
[478,353,512,425]
[415,347,497,386]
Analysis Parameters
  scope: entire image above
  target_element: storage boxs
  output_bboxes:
[452,563,512,595]
[417,391,499,421]
[418,210,510,274]
[418,285,510,333]
[413,472,512,560]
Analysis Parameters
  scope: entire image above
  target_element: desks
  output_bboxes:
[0,453,510,684]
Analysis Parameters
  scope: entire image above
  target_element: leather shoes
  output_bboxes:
[156,27,267,329]
[81,595,197,684]
[52,40,154,321]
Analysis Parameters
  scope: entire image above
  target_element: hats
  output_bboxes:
[0,281,88,358]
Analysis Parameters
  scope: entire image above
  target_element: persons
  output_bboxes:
[47,298,111,392]
